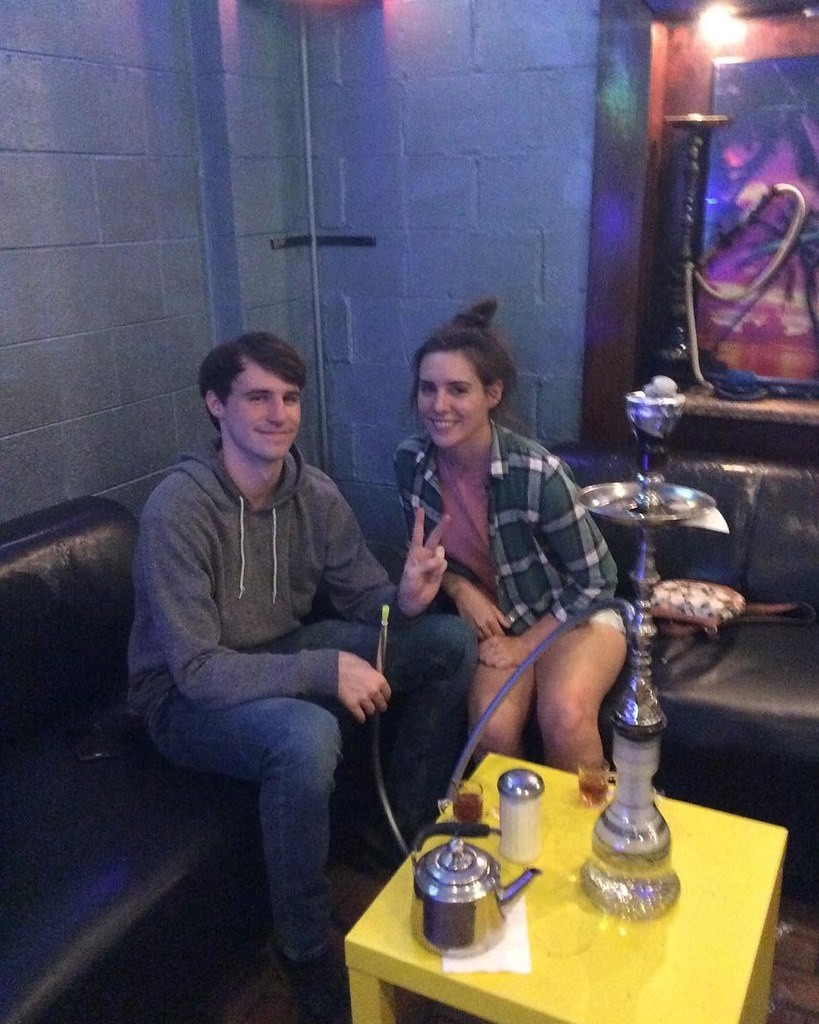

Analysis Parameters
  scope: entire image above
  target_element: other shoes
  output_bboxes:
[283,947,349,1024]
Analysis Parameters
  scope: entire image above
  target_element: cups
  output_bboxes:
[576,755,620,805]
[448,778,484,821]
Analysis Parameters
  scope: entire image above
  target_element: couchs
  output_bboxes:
[0,497,256,1023]
[427,448,819,775]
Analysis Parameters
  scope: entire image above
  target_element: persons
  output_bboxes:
[129,332,476,1024]
[391,292,626,772]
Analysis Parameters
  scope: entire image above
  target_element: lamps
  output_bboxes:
[443,376,718,920]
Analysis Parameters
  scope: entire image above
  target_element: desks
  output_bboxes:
[344,751,789,1024]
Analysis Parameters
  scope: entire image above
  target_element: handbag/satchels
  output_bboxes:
[650,580,747,635]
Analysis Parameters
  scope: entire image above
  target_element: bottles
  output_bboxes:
[497,768,544,865]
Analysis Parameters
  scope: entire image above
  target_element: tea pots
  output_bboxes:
[409,821,543,956]
